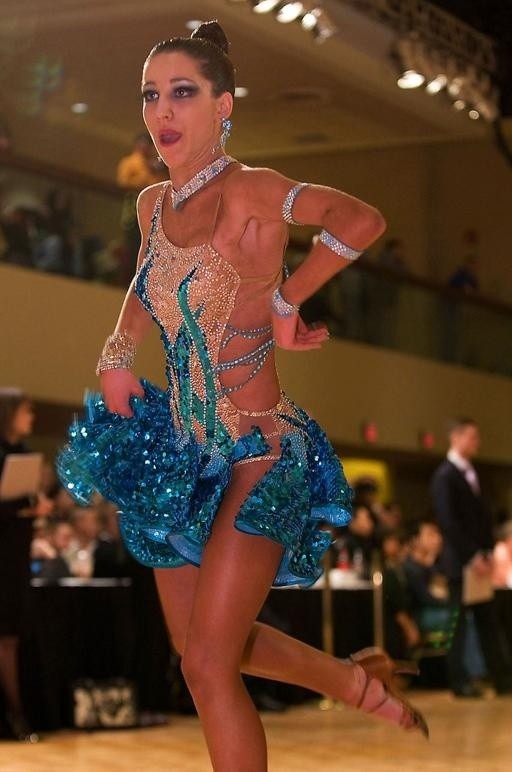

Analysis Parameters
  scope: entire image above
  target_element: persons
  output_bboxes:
[52,14,436,771]
[425,412,511,699]
[299,287,347,338]
[115,133,165,282]
[1,183,90,277]
[25,479,134,582]
[329,471,453,690]
[0,384,54,744]
[361,236,408,348]
[433,251,481,360]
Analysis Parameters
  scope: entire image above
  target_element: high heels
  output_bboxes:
[349,648,430,740]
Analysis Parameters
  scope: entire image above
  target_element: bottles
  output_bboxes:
[335,545,366,579]
